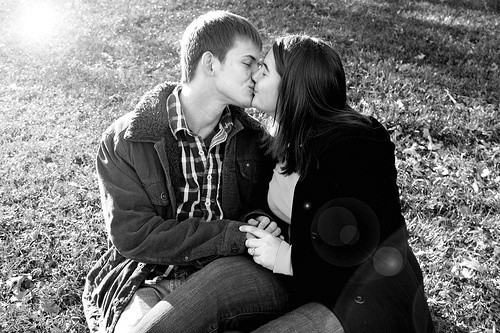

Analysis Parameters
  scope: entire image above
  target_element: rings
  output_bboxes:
[249,247,256,255]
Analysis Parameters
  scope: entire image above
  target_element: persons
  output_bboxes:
[127,34,437,333]
[81,13,276,333]
